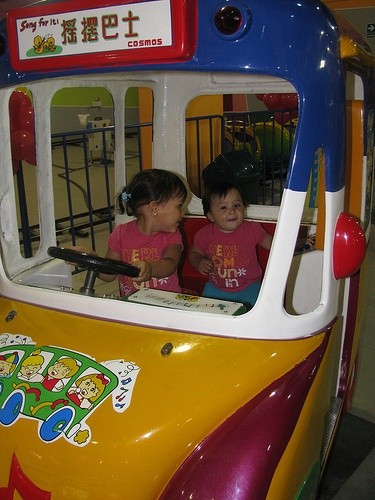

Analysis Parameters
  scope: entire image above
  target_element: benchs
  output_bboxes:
[176,215,317,314]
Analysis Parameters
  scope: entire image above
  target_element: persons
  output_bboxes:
[62,168,187,300]
[188,180,319,312]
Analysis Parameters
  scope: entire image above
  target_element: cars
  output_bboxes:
[1,0,369,500]
